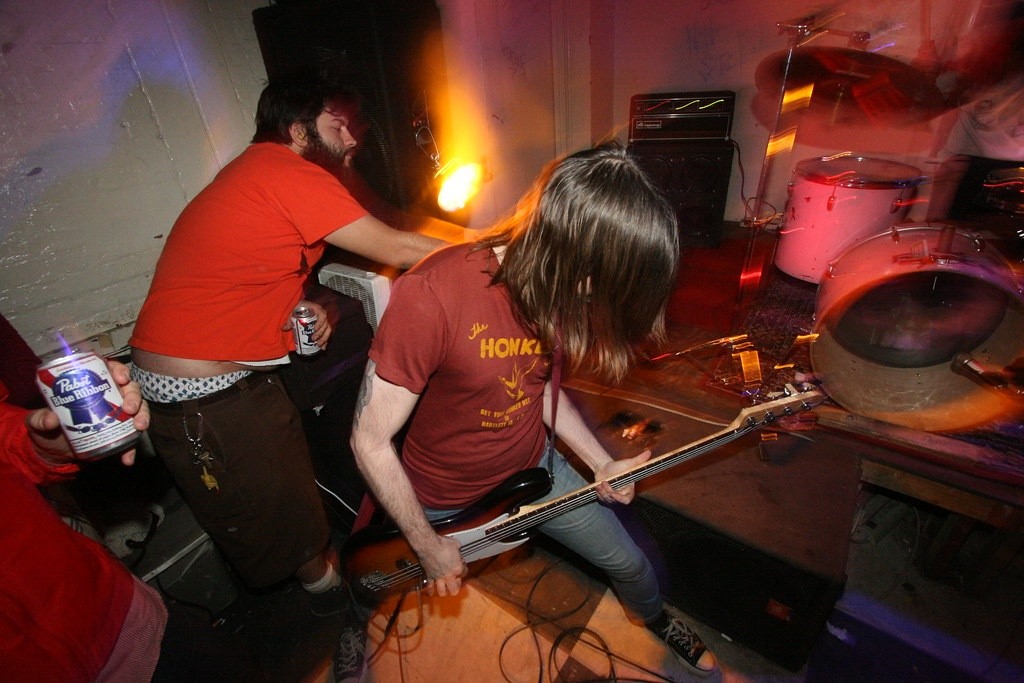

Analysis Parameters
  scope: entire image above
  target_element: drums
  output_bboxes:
[811,220,1024,431]
[774,150,922,284]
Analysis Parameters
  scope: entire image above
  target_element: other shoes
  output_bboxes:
[311,581,361,618]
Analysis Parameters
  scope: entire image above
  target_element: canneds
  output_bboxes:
[291,306,323,356]
[34,352,142,463]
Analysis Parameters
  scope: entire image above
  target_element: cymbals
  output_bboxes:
[756,45,943,128]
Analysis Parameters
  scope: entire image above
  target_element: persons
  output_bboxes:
[349,144,718,676]
[127,76,453,617]
[0,361,196,682]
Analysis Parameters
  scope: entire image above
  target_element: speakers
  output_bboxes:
[251,0,469,229]
[627,139,733,249]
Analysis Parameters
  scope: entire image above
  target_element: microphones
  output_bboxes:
[953,351,1024,396]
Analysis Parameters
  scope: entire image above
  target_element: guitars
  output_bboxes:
[340,382,824,610]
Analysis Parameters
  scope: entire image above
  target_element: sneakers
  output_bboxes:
[645,609,717,677]
[334,621,367,683]
